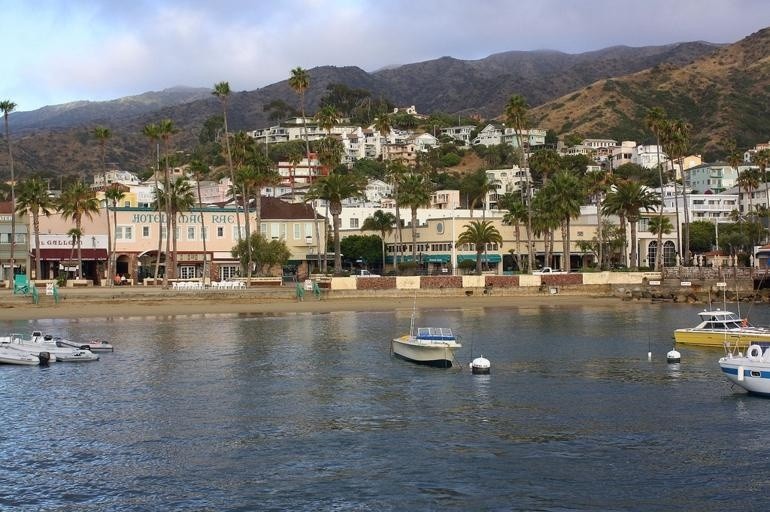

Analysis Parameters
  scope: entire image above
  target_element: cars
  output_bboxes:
[349,269,380,278]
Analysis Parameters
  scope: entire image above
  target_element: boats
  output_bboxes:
[391,292,462,369]
[1,332,114,365]
[718,345,770,395]
[673,276,769,347]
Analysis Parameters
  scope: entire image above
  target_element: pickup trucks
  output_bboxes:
[532,267,566,274]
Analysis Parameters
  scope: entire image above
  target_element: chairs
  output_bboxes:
[172,280,248,292]
